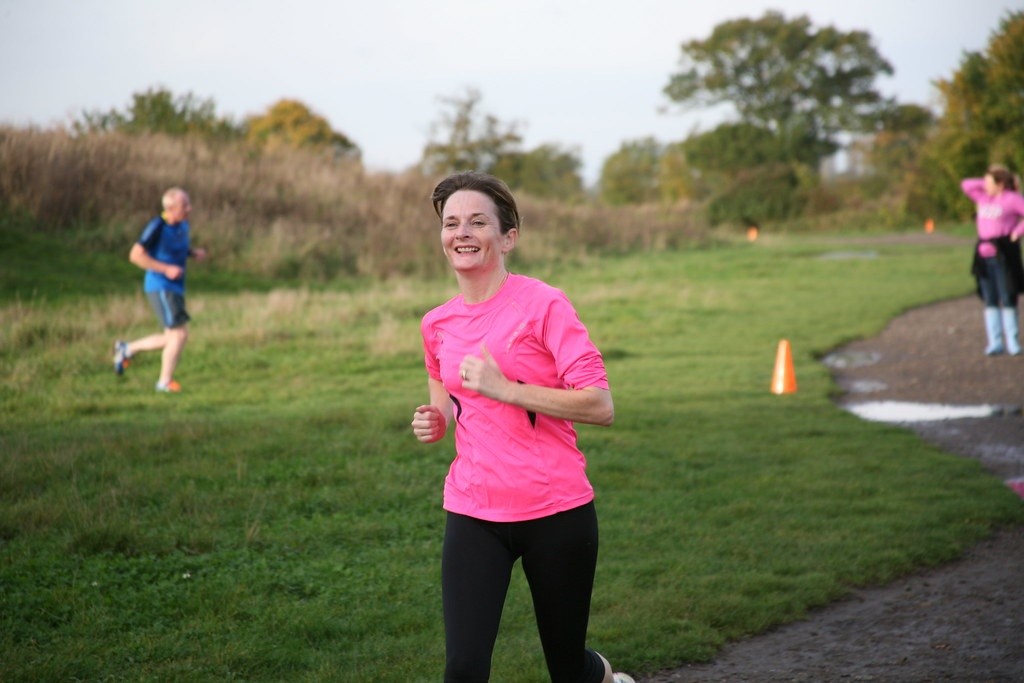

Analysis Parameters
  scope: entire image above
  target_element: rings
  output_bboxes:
[462,369,467,380]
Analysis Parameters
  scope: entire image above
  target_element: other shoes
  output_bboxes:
[619,672,635,683]
[155,380,190,395]
[113,340,128,376]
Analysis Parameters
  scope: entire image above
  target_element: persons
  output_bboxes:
[115,189,204,393]
[412,173,633,683]
[961,166,1024,356]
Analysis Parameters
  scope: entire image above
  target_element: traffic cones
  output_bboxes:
[767,338,797,395]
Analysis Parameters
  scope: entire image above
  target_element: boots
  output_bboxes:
[1001,307,1021,356]
[982,307,1006,354]
